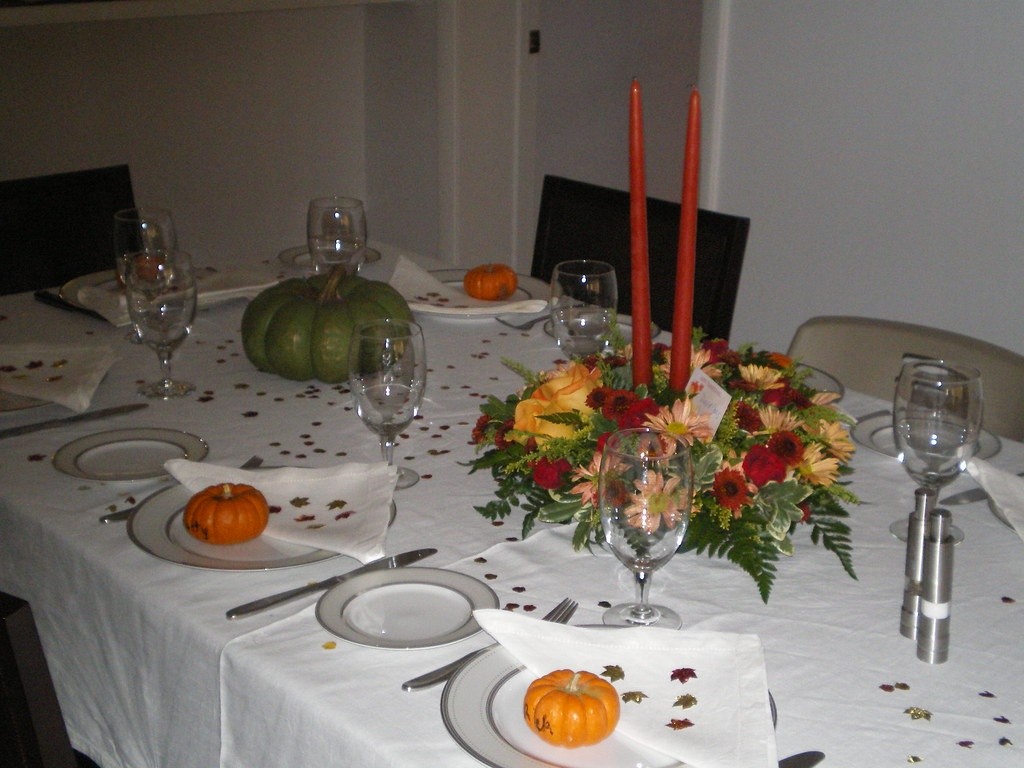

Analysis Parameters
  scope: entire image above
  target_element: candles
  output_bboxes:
[669,84,702,390]
[629,76,654,387]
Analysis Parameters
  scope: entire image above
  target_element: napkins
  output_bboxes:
[965,455,1024,542]
[76,257,280,327]
[163,456,401,564]
[472,607,779,768]
[0,340,124,414]
[389,254,547,315]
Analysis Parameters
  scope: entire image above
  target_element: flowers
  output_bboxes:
[455,322,876,604]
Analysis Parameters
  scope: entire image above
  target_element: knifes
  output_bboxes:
[0,403,149,441]
[938,472,1024,504]
[224,548,438,619]
[779,750,825,768]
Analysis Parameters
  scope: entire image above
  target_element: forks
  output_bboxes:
[99,455,264,524]
[399,597,580,693]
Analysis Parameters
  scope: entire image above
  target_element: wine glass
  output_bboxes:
[886,359,984,546]
[122,248,199,400]
[347,319,429,491]
[597,426,695,631]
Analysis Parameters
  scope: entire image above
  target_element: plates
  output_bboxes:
[125,481,397,569]
[849,408,1004,471]
[277,243,382,269]
[52,427,210,488]
[314,566,500,651]
[398,268,551,320]
[544,312,661,345]
[794,362,845,405]
[58,269,122,313]
[439,639,778,768]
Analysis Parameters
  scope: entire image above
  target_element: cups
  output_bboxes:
[112,207,177,290]
[550,259,618,361]
[306,196,368,274]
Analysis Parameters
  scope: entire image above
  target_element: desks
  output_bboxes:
[0,237,1024,768]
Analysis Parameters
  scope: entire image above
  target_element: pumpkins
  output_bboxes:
[524,669,620,748]
[463,263,516,301]
[751,351,793,370]
[183,482,270,545]
[242,264,412,384]
[116,255,173,286]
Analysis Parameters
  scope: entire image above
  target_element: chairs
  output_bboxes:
[0,164,146,296]
[528,174,751,344]
[787,315,1024,446]
